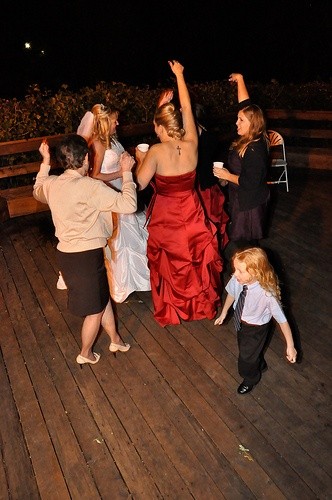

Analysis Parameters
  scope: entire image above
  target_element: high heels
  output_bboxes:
[109,342,130,358]
[76,351,101,369]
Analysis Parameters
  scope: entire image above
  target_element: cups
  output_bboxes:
[137,144,150,152]
[213,162,224,168]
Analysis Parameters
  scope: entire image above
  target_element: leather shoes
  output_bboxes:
[237,384,253,393]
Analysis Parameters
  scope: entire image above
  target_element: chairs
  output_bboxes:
[265,130,289,193]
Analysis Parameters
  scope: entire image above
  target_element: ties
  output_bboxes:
[233,285,248,332]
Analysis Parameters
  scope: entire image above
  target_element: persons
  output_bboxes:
[214,248,297,394]
[33,60,272,367]
